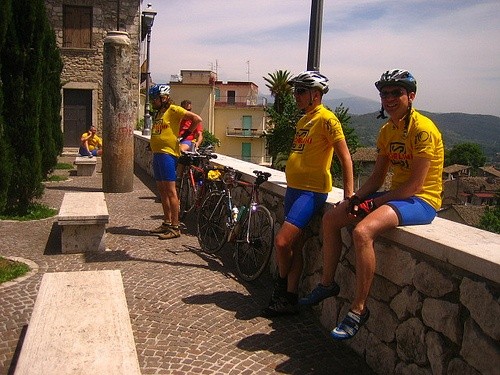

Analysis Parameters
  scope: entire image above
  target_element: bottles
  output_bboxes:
[197,180,203,198]
[233,205,239,223]
[238,204,246,222]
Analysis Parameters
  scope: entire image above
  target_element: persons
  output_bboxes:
[176,99,203,198]
[299,69,444,338]
[149,84,202,240]
[80,126,102,172]
[261,72,354,314]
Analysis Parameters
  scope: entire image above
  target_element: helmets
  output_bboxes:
[375,69,417,91]
[148,84,170,95]
[288,71,329,93]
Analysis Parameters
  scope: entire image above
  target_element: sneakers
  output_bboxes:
[158,225,180,238]
[300,281,340,304]
[268,296,302,313]
[272,276,287,301]
[150,222,172,233]
[331,305,370,339]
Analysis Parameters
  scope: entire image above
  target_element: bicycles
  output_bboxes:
[195,164,275,284]
[174,144,231,239]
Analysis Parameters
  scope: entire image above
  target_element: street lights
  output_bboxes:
[141,3,157,136]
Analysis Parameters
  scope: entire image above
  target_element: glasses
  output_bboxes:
[379,89,404,97]
[295,87,308,93]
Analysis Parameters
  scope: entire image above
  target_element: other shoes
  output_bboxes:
[88,151,93,158]
